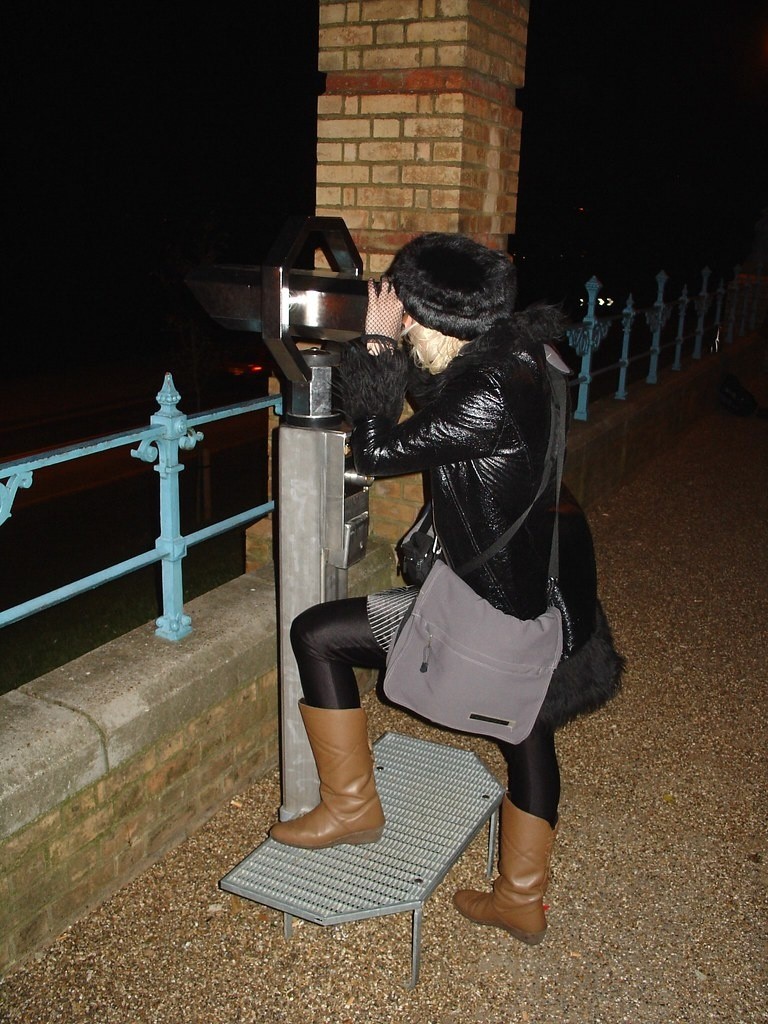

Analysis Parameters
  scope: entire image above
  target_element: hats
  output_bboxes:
[383,232,516,342]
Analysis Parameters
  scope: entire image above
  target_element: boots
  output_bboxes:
[269,699,386,850]
[452,791,560,945]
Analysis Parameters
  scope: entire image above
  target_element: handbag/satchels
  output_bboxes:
[375,560,567,745]
[396,503,444,590]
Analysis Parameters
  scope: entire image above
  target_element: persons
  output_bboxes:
[271,230,626,946]
